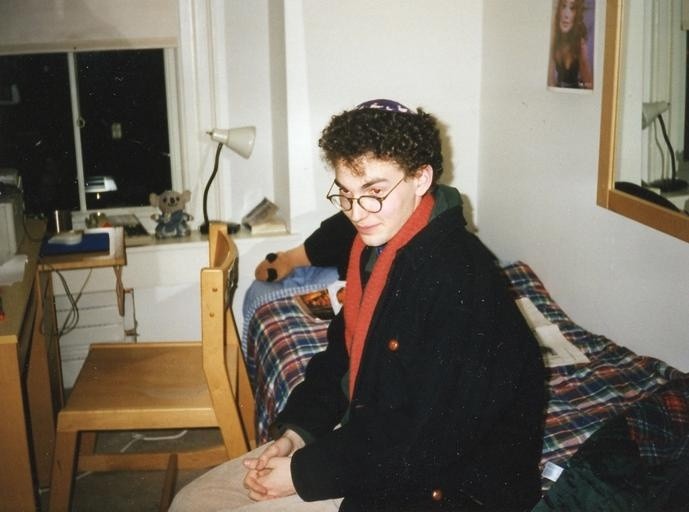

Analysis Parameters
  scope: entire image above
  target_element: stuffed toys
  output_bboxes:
[149,187,194,242]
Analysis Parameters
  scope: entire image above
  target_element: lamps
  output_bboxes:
[193,121,261,238]
[638,97,689,195]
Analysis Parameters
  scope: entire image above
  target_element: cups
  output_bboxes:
[48,211,72,233]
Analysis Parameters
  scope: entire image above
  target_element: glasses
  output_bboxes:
[325,177,405,214]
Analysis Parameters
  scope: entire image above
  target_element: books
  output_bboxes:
[513,295,592,372]
[290,287,337,325]
[241,197,288,235]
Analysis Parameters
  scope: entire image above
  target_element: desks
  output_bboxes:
[2,210,65,509]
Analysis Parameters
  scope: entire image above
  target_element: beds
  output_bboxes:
[234,249,688,508]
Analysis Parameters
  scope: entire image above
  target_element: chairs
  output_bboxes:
[43,221,270,512]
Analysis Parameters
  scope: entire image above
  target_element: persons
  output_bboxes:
[170,104,548,512]
[546,1,594,90]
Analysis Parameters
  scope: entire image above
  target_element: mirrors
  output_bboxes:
[593,0,689,246]
